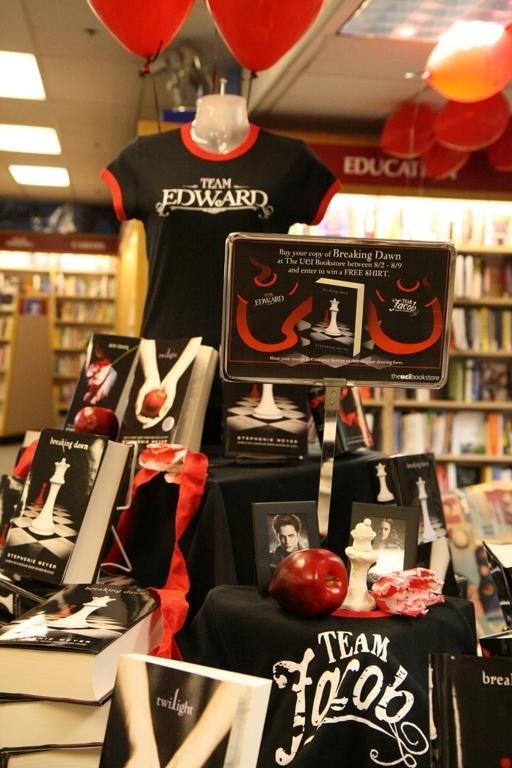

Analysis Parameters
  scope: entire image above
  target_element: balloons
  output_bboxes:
[379,20,512,180]
[87,0,324,73]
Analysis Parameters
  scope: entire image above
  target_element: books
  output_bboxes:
[366,452,449,546]
[0,332,273,768]
[225,381,307,457]
[308,386,369,458]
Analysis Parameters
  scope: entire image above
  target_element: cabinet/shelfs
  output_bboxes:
[6,265,119,430]
[2,314,64,447]
[348,246,510,492]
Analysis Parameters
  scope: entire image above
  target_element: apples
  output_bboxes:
[267,547,348,620]
[74,406,119,441]
[142,389,166,418]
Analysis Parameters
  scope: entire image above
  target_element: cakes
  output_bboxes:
[371,566,445,621]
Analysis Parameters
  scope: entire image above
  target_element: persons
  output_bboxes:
[269,514,306,568]
[100,94,344,459]
[373,517,401,551]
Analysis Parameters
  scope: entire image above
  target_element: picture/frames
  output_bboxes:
[255,501,420,594]
